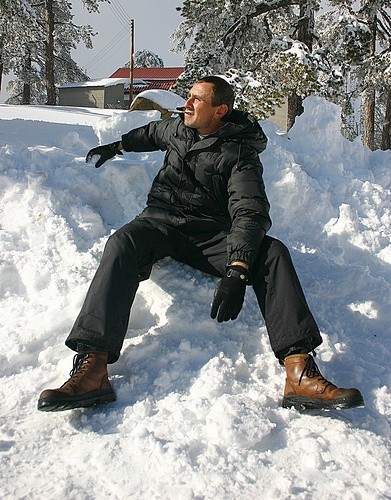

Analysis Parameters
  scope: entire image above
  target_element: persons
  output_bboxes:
[35,75,365,413]
